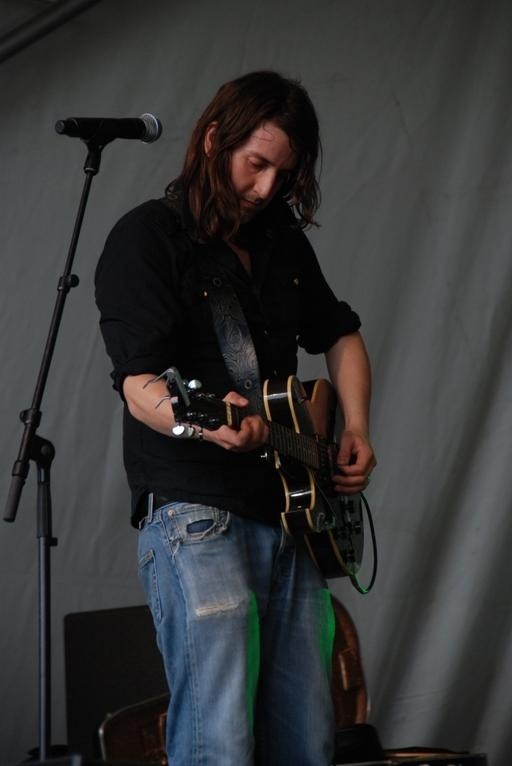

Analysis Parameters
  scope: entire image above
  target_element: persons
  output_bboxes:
[93,70,377,765]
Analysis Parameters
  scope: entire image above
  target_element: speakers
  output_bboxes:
[63,604,171,766]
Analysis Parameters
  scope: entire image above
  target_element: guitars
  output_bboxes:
[165,366,364,578]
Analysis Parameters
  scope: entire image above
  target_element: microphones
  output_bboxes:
[54,112,163,144]
